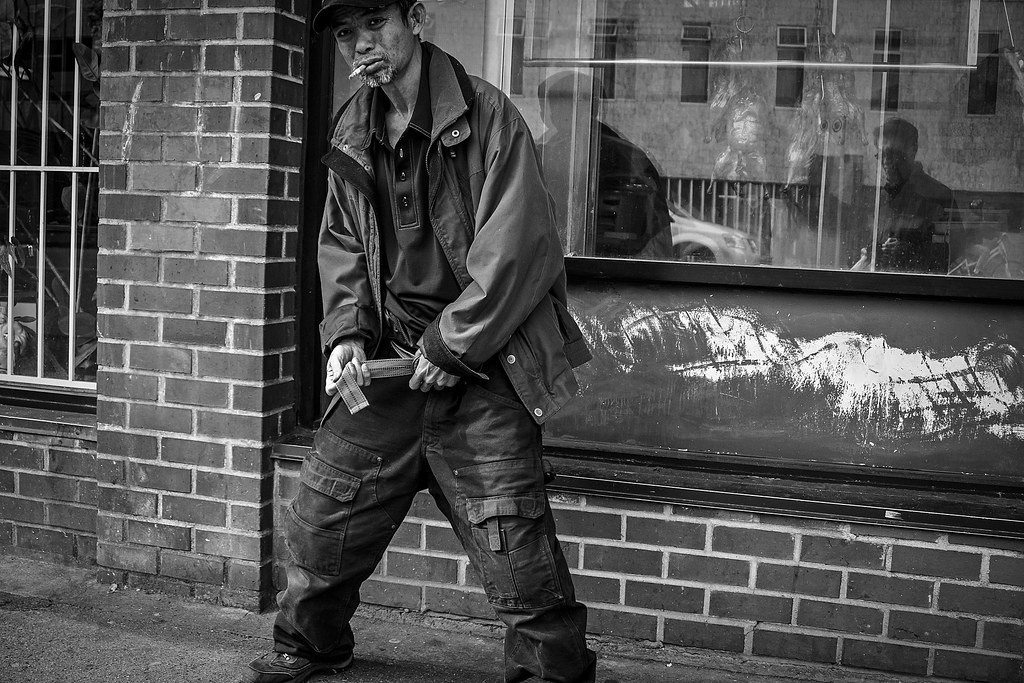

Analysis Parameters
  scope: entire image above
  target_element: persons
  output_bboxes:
[531,67,674,260]
[248,1,597,683]
[846,117,965,275]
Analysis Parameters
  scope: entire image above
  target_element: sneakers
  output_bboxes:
[239,649,355,683]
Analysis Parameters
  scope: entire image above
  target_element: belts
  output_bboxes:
[333,340,420,415]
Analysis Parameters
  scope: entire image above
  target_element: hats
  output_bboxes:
[312,0,386,34]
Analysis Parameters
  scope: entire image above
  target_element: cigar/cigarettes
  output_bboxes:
[348,64,366,79]
